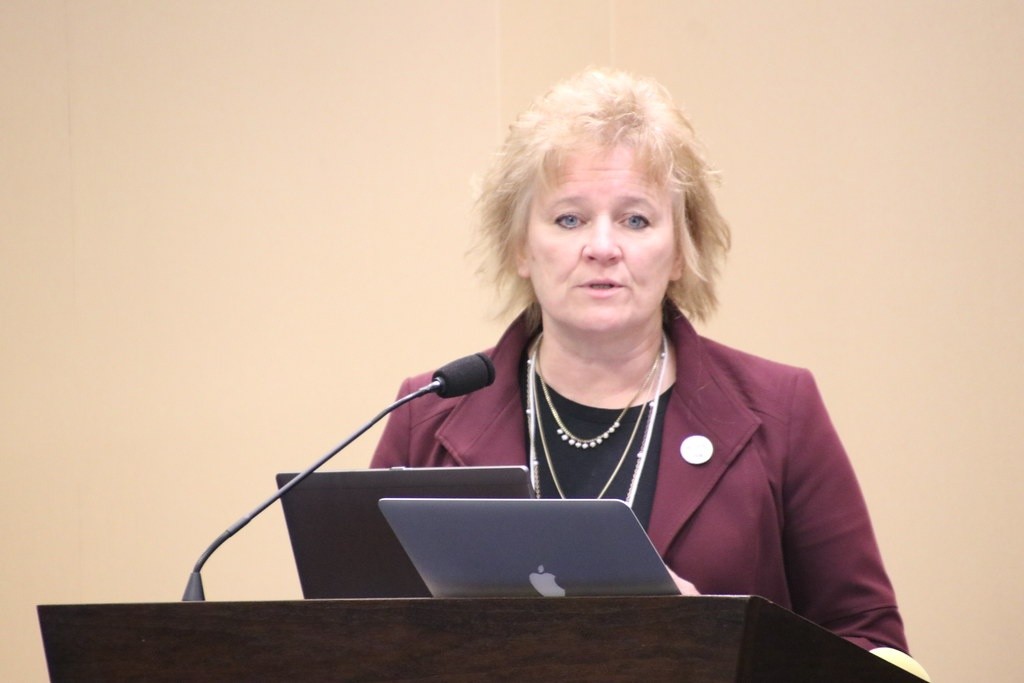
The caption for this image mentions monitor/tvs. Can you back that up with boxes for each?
[275,465,537,599]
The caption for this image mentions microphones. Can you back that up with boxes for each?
[181,352,498,604]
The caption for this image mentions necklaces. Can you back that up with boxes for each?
[525,329,669,506]
[537,346,661,449]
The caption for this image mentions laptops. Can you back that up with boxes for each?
[377,497,684,599]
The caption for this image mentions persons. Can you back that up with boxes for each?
[369,70,930,683]
